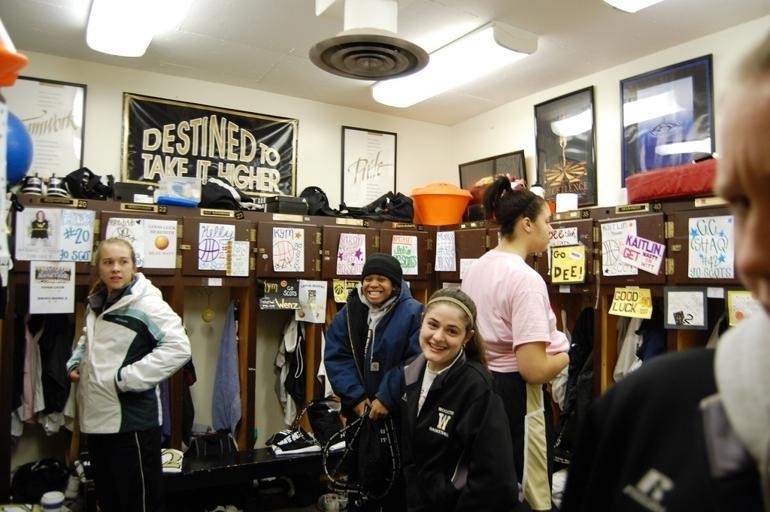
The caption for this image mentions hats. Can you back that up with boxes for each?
[362,253,403,288]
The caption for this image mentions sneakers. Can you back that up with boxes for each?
[16,176,42,197]
[48,178,72,193]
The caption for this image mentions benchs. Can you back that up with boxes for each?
[169,435,348,512]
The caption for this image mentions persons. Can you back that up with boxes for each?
[25,210,52,249]
[561,34,770,511]
[65,238,192,512]
[324,254,427,512]
[460,176,569,512]
[404,287,518,512]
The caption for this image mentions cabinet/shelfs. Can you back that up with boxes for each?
[431,195,764,441]
[3,194,431,512]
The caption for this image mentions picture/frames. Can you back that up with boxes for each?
[619,53,716,189]
[458,149,527,189]
[340,125,397,210]
[121,91,300,199]
[0,75,87,178]
[533,85,598,209]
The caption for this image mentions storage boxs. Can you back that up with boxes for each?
[113,182,154,204]
[265,196,307,215]
[157,174,202,208]
